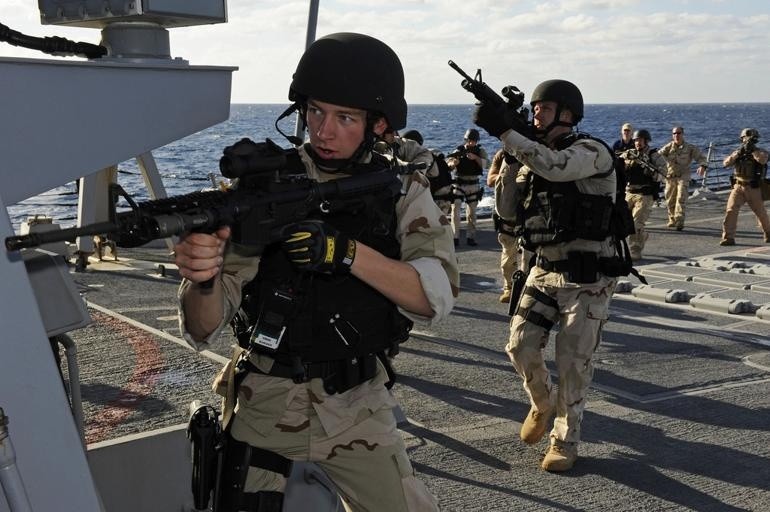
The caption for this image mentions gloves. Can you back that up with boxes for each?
[472,104,511,140]
[279,219,356,278]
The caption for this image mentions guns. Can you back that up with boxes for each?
[443,145,481,164]
[449,60,547,164]
[5,140,403,301]
[740,134,759,161]
[624,147,669,179]
[188,399,218,511]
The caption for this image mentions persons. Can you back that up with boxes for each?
[473,77,622,472]
[612,122,635,154]
[173,33,459,511]
[400,130,452,223]
[487,147,532,301]
[620,129,667,263]
[658,126,709,232]
[447,129,489,247]
[720,127,770,245]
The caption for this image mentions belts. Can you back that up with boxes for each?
[246,360,342,383]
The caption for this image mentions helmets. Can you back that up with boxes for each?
[631,130,652,142]
[740,127,762,140]
[403,129,424,145]
[289,32,407,132]
[530,80,584,119]
[463,128,481,142]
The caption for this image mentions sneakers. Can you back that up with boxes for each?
[541,436,577,471]
[763,231,770,245]
[521,406,555,443]
[667,219,685,231]
[500,287,511,304]
[630,250,641,261]
[719,239,736,247]
